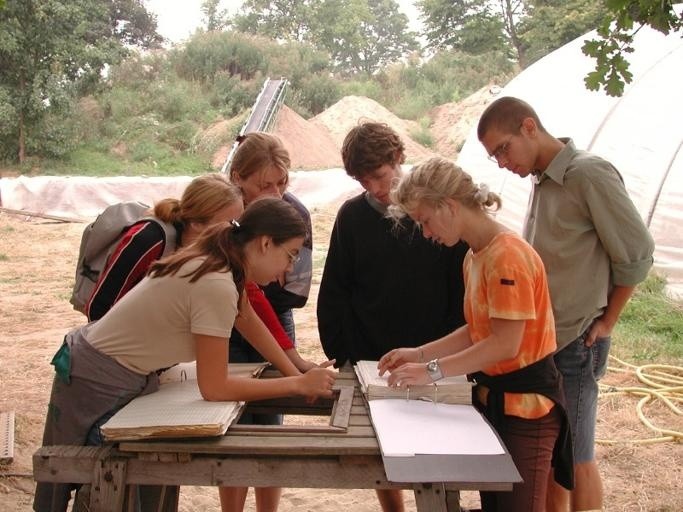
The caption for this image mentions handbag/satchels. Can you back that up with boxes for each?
[68,199,178,316]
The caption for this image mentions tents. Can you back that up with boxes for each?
[456,4,682,308]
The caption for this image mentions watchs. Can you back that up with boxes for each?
[426,358,443,382]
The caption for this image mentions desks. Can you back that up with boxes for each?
[32,367,514,512]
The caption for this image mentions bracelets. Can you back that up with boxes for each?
[416,345,425,361]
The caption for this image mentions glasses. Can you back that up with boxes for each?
[485,121,526,164]
[274,240,302,267]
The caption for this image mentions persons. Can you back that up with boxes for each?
[218,134,313,512]
[376,157,575,512]
[317,117,468,511]
[85,174,337,373]
[32,197,336,512]
[477,97,656,512]
